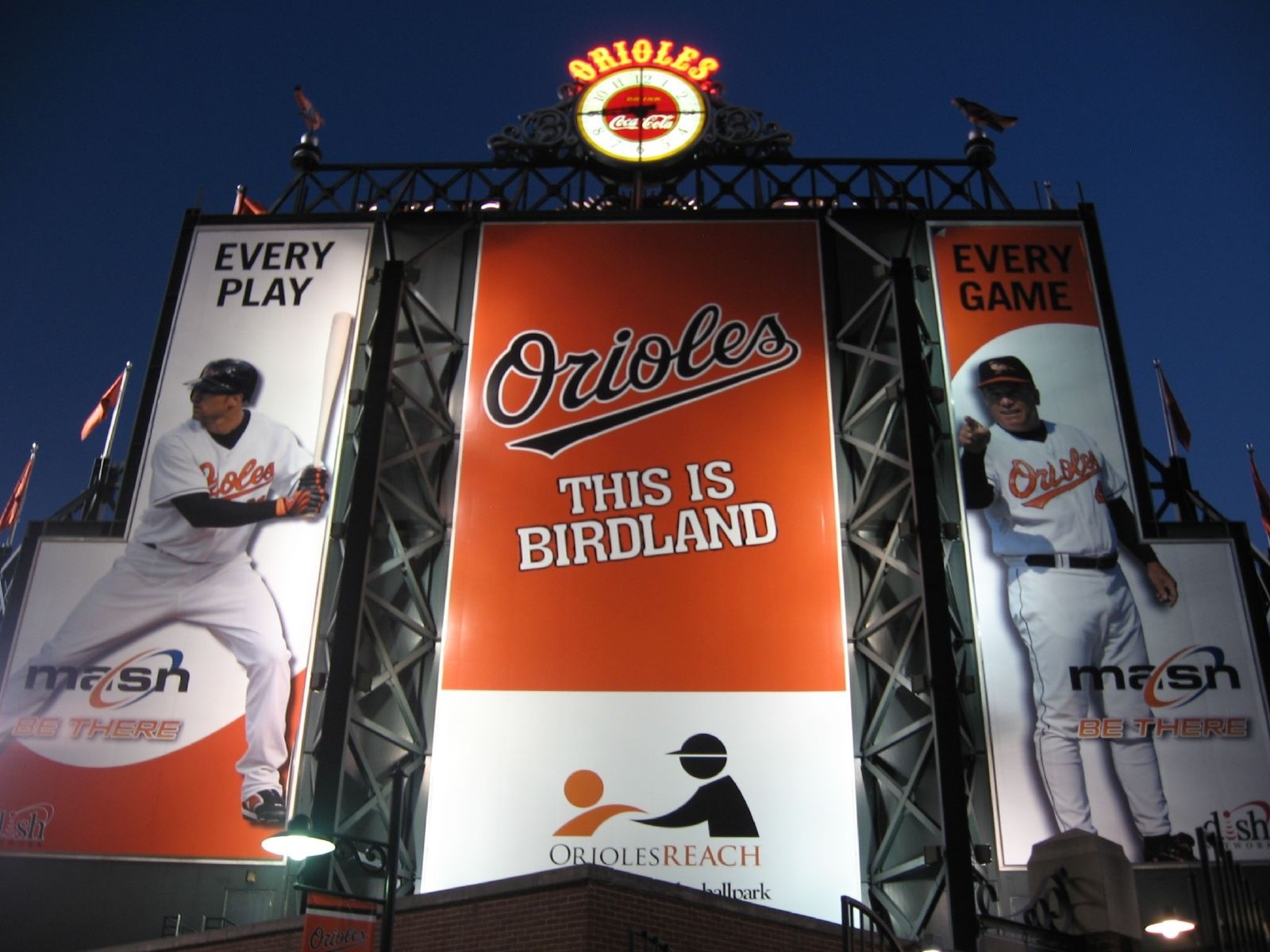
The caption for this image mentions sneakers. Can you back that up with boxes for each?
[240,788,286,824]
[1142,833,1199,865]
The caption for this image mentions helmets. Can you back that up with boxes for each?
[182,358,258,404]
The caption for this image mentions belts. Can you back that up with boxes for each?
[1024,550,1118,570]
[145,542,158,549]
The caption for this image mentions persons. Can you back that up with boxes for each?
[0,359,327,824]
[955,355,1198,864]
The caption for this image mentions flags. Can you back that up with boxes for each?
[1248,457,1270,540]
[0,460,33,531]
[81,373,123,440]
[1160,373,1192,452]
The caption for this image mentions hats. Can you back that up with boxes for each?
[976,355,1033,390]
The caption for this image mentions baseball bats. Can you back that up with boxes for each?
[304,314,354,519]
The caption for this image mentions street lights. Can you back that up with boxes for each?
[260,766,407,952]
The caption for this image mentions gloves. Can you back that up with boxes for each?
[277,467,330,520]
